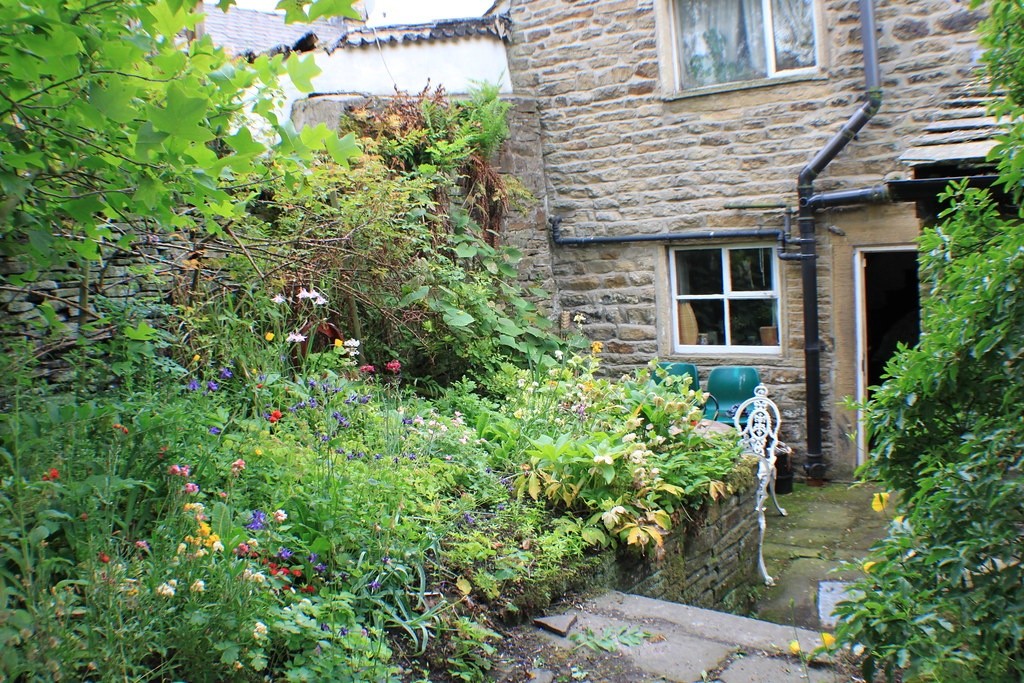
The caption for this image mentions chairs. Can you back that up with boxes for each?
[650,363,760,426]
[732,382,788,587]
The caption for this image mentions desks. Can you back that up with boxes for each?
[694,418,735,438]
